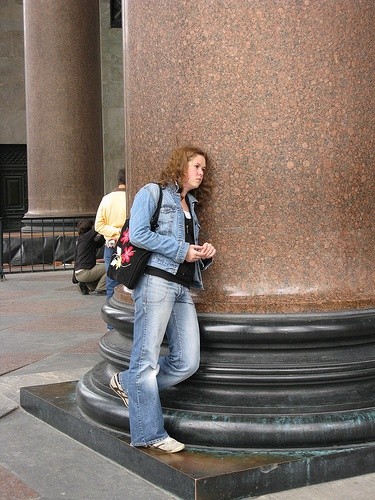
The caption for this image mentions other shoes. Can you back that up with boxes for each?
[97,290,107,296]
[79,283,89,296]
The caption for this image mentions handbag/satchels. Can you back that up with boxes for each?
[106,181,163,290]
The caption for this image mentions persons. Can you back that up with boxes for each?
[94,167,126,333]
[75,218,108,296]
[109,147,217,453]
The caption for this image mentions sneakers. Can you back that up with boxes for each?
[148,435,186,454]
[109,372,132,409]
